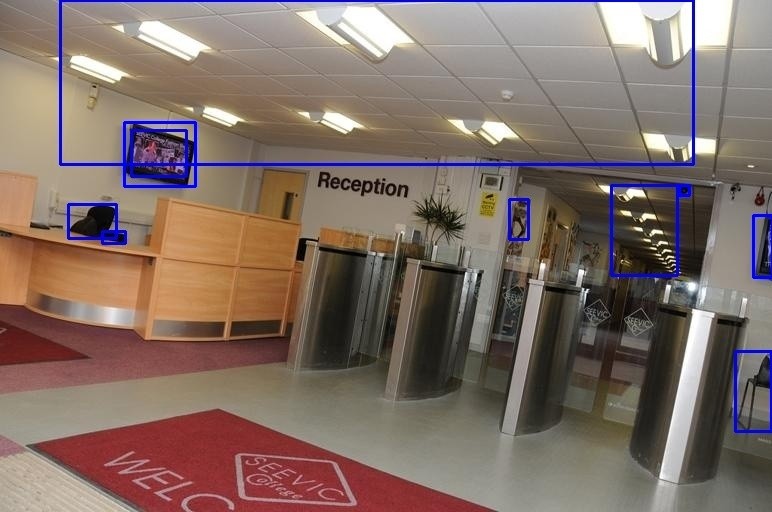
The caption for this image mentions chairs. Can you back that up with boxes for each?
[736,351,772,432]
[68,205,116,239]
[102,232,125,243]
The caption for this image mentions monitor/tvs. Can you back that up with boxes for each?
[754,215,772,278]
[125,123,196,187]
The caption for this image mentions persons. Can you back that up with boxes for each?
[509,199,528,239]
[131,131,187,176]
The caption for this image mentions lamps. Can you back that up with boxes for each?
[187,104,247,129]
[443,118,519,146]
[596,2,692,69]
[299,110,363,134]
[612,185,678,275]
[642,131,693,163]
[60,54,134,83]
[295,5,412,64]
[112,21,212,65]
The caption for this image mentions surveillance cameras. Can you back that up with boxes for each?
[679,185,689,195]
